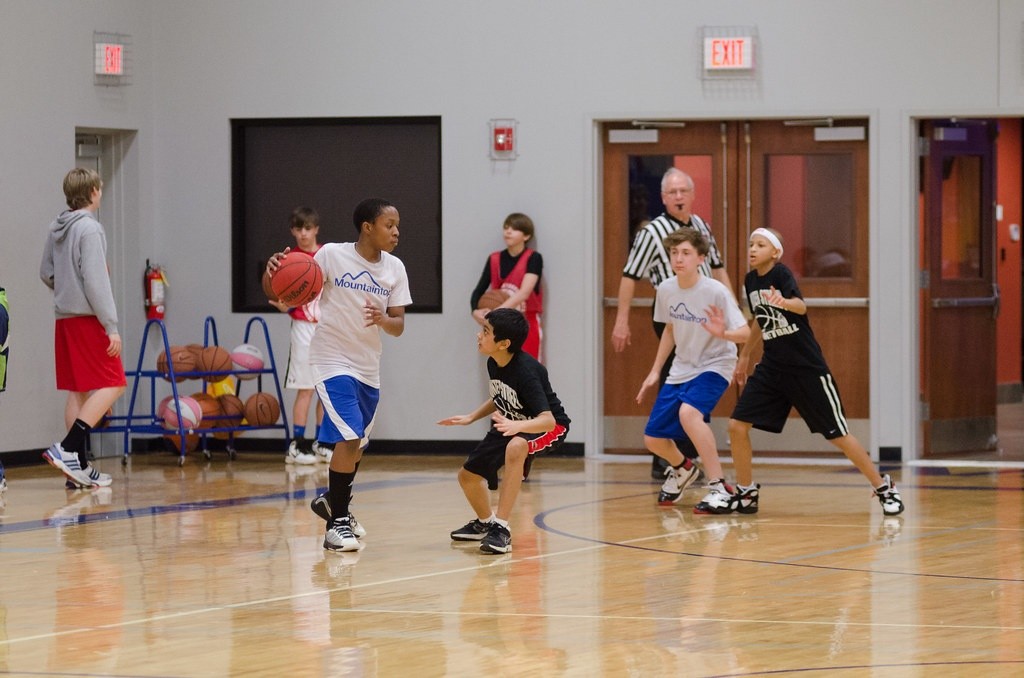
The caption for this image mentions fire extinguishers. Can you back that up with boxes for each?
[146,264,166,320]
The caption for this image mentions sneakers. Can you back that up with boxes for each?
[734,483,760,514]
[44,444,112,490]
[692,479,739,515]
[658,506,700,546]
[481,553,513,586]
[285,463,330,486]
[284,439,333,465]
[479,522,513,554]
[731,512,759,543]
[692,513,732,543]
[450,519,492,541]
[657,459,700,506]
[877,516,904,548]
[49,487,113,525]
[310,494,366,554]
[324,541,366,580]
[872,474,905,516]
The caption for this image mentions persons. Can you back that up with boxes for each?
[0,287,10,496]
[263,198,413,552]
[470,213,545,363]
[268,205,335,465]
[437,308,571,552]
[39,167,129,491]
[799,247,851,276]
[611,169,740,483]
[636,226,751,514]
[709,228,904,517]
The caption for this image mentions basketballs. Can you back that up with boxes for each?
[216,395,245,428]
[245,393,280,426]
[184,344,203,380]
[189,393,219,428]
[262,269,280,302]
[158,395,203,430]
[92,406,113,428]
[157,346,195,383]
[167,432,199,454]
[230,344,264,380]
[478,290,510,311]
[197,346,233,383]
[270,252,324,307]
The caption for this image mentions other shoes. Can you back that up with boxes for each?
[651,455,705,481]
